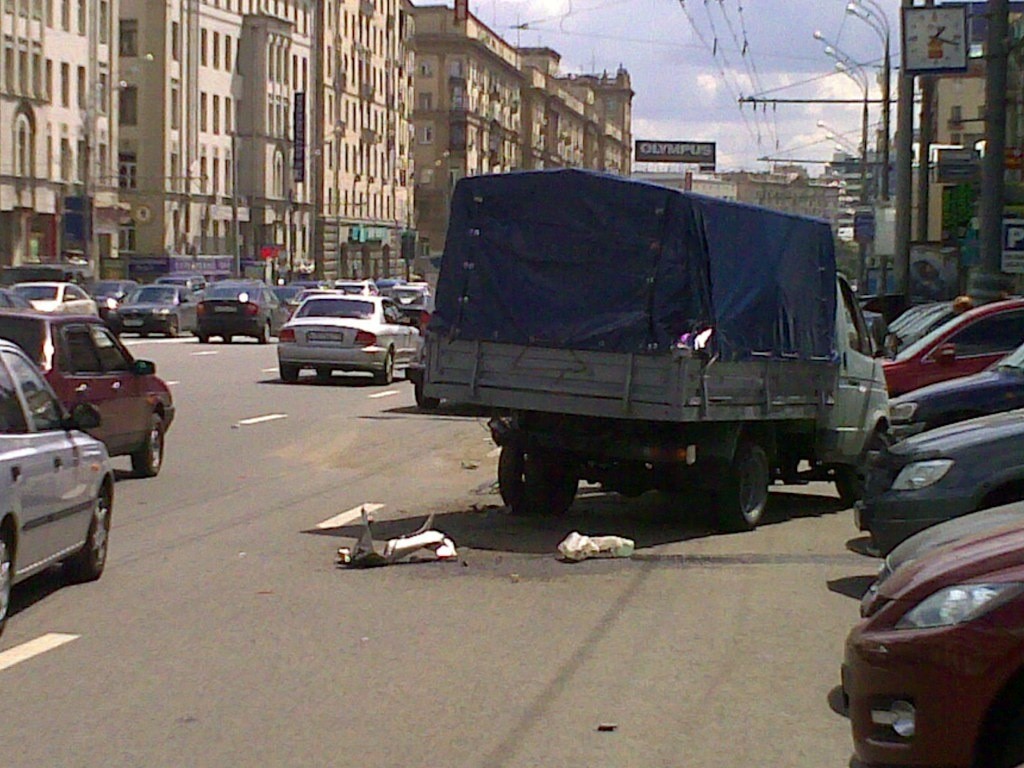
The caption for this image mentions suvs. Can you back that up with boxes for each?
[155,273,208,302]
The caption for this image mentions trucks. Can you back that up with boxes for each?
[422,169,893,531]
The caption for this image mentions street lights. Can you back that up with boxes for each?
[845,1,890,299]
[812,31,869,295]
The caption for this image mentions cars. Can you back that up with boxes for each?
[85,280,139,324]
[195,280,293,344]
[877,298,1024,398]
[270,277,430,331]
[0,310,176,478]
[0,288,35,312]
[8,279,100,315]
[0,340,116,640]
[882,344,1024,445]
[845,289,1000,360]
[855,409,1024,560]
[108,285,200,337]
[278,294,425,387]
[828,501,1023,768]
